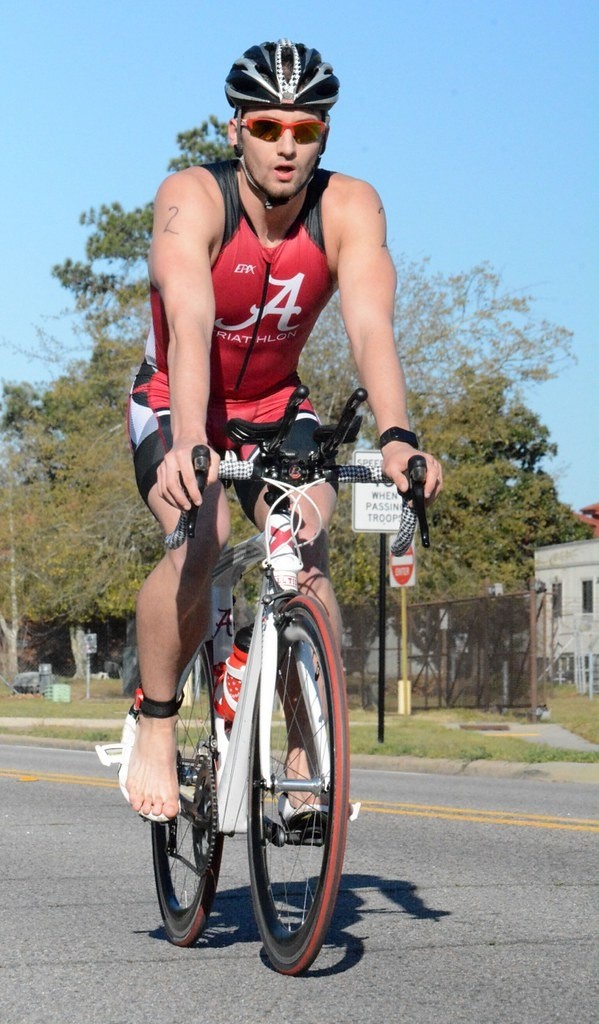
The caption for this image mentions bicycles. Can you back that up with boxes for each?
[94,386,432,976]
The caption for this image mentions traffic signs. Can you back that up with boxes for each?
[388,534,416,588]
[84,633,97,654]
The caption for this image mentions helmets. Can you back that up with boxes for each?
[224,38,341,111]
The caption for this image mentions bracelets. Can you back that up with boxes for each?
[137,687,185,718]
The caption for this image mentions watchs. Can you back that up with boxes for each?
[375,426,421,453]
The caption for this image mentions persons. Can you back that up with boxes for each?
[117,35,443,822]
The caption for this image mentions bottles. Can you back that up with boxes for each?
[214,624,254,720]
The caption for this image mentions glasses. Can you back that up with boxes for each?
[235,115,326,145]
